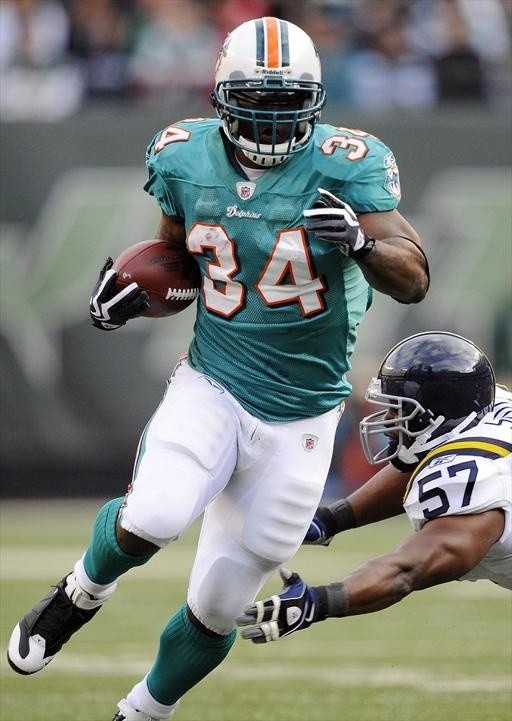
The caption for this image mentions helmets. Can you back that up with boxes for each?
[375,330,496,472]
[206,14,327,171]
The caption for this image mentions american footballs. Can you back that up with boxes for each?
[111,239,199,320]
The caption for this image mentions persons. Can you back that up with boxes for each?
[7,14,433,720]
[232,327,512,647]
[0,1,511,123]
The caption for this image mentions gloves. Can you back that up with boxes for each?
[299,187,375,264]
[87,253,154,335]
[234,566,329,647]
[299,506,339,547]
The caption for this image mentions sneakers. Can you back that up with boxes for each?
[6,567,108,677]
